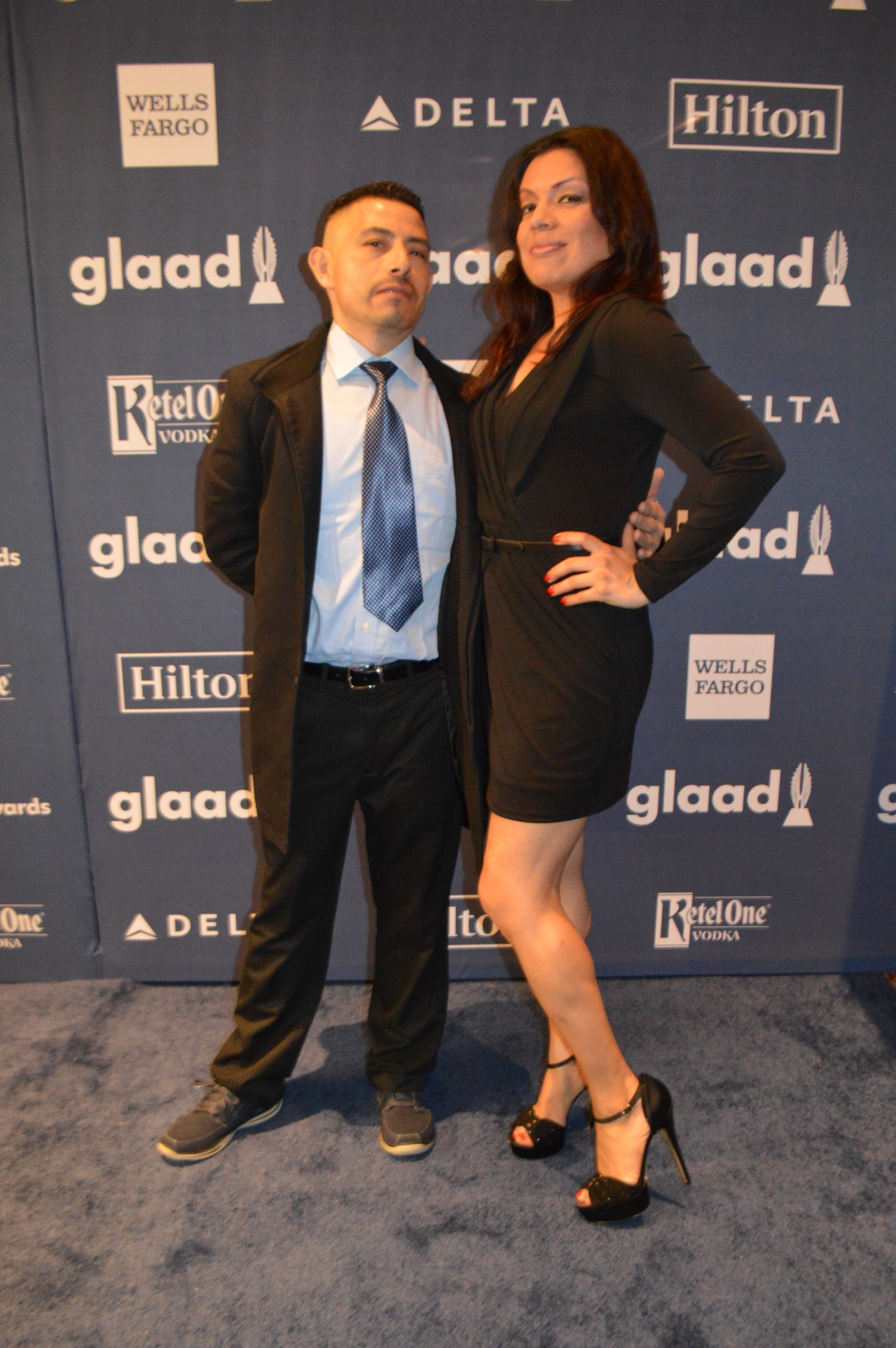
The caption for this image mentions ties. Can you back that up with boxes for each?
[362,358,428,629]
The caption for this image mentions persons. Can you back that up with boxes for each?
[154,179,666,1165]
[457,123,790,1221]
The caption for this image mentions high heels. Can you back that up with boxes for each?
[508,1048,589,1160]
[574,1072,693,1222]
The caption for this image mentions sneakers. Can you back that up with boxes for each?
[154,1073,290,1164]
[376,1081,435,1162]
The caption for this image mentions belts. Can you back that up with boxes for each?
[297,659,448,691]
[477,533,633,547]
